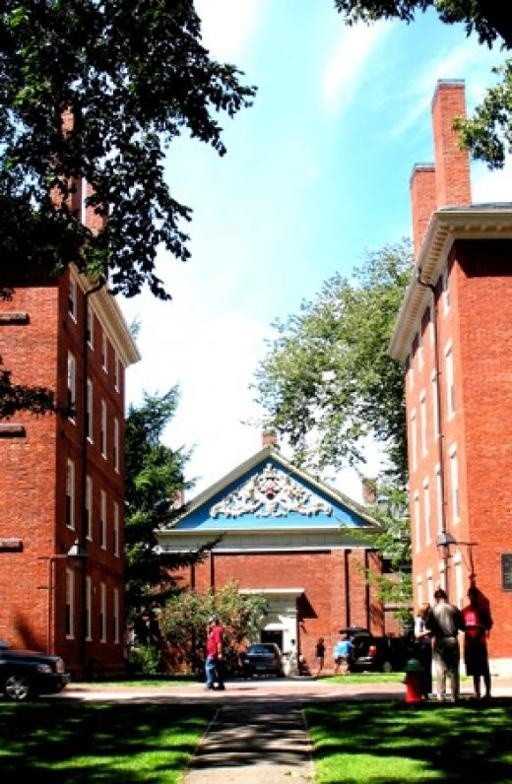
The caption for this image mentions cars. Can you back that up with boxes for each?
[243,643,288,678]
[339,627,420,673]
[0,641,71,702]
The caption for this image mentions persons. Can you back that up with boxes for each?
[333,633,356,673]
[313,637,324,679]
[414,586,493,701]
[204,615,225,689]
